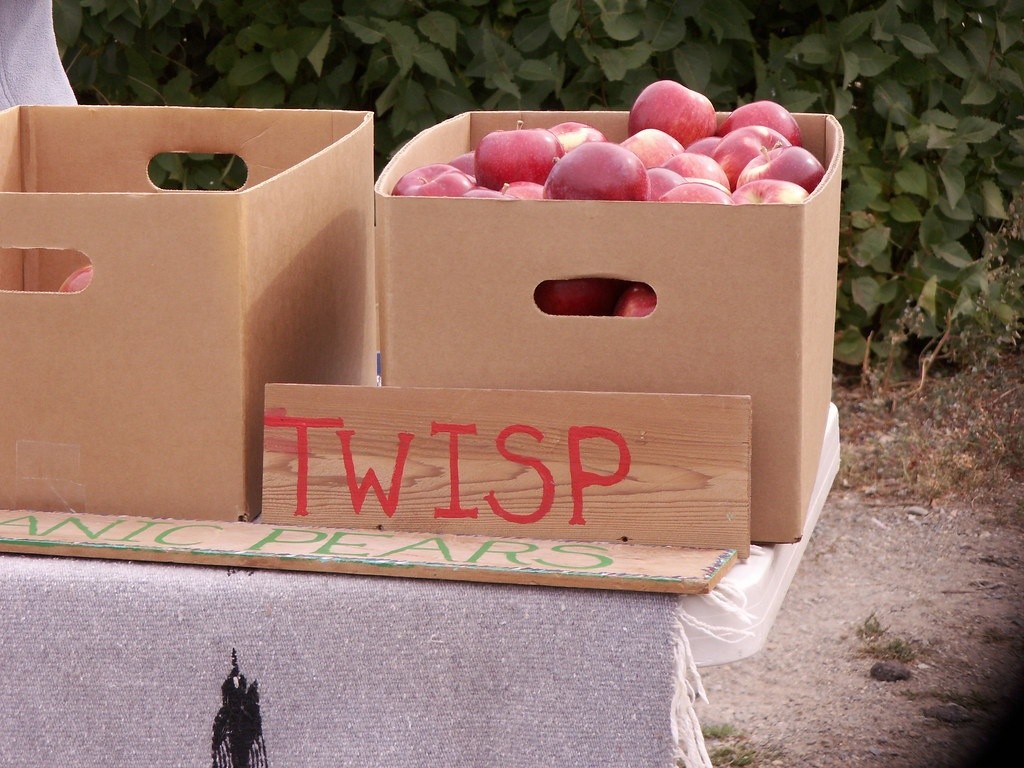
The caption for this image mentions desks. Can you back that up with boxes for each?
[1,403,845,768]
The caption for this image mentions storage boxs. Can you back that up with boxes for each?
[374,111,845,543]
[0,103,374,524]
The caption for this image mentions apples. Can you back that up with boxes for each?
[388,80,824,317]
[57,265,93,294]
